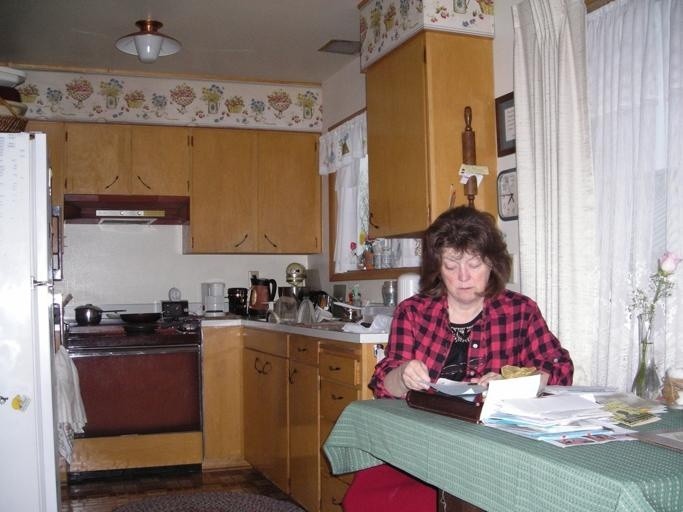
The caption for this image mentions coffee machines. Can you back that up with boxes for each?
[199,281,225,319]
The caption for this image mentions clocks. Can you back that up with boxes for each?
[496,168,520,221]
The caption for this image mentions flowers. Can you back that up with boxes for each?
[626,250,682,321]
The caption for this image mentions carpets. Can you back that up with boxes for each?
[110,490,308,512]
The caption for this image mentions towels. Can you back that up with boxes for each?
[54,346,87,467]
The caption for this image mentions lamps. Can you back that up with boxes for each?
[113,17,181,64]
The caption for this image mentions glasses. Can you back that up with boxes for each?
[440,357,485,377]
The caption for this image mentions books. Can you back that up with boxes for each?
[406,372,683,452]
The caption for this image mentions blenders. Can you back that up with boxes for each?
[278,262,311,308]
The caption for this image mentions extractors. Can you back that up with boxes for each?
[63,199,187,227]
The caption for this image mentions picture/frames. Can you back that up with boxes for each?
[494,91,515,158]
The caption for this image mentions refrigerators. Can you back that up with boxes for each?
[0,133,62,512]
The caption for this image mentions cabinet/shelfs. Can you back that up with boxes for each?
[242,327,288,495]
[182,128,321,255]
[25,121,64,254]
[67,124,189,196]
[319,339,362,512]
[288,335,321,512]
[365,31,498,242]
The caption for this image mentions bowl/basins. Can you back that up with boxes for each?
[331,300,365,322]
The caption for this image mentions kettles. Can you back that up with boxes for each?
[248,274,277,315]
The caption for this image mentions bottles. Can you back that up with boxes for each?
[365,249,374,270]
[227,286,248,315]
[373,248,383,269]
[381,247,392,268]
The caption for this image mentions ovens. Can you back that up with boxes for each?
[65,340,202,439]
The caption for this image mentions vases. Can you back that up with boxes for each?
[630,314,661,400]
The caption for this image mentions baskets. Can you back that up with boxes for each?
[0,98,28,132]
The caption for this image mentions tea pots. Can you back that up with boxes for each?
[174,311,201,333]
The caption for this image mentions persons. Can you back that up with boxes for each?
[340,204,575,511]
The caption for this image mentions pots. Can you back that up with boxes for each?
[74,303,127,325]
[119,311,164,322]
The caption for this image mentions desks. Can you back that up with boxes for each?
[321,385,682,512]
